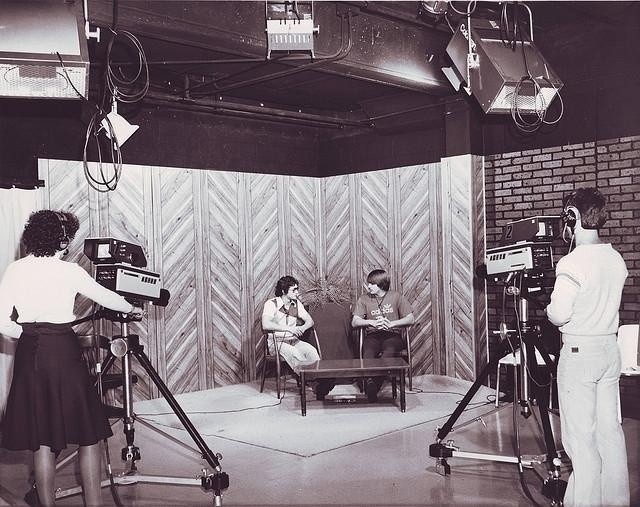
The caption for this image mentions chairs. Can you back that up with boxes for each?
[616,322,640,426]
[493,319,564,412]
[359,325,412,389]
[260,319,323,399]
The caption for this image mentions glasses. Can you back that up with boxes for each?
[288,286,299,293]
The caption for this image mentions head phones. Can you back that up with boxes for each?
[560,189,582,232]
[53,209,70,251]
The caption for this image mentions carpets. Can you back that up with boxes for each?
[117,370,506,461]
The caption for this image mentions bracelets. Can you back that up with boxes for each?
[131,305,135,312]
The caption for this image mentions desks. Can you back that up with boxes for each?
[300,356,410,416]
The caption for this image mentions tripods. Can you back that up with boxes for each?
[24,325,230,505]
[428,316,569,506]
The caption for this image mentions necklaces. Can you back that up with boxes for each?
[373,292,386,318]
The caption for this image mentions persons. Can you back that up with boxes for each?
[351,269,416,402]
[544,186,633,507]
[0,208,146,506]
[261,275,337,401]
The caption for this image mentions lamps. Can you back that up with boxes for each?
[264,1,320,61]
[417,1,449,21]
[95,86,142,152]
